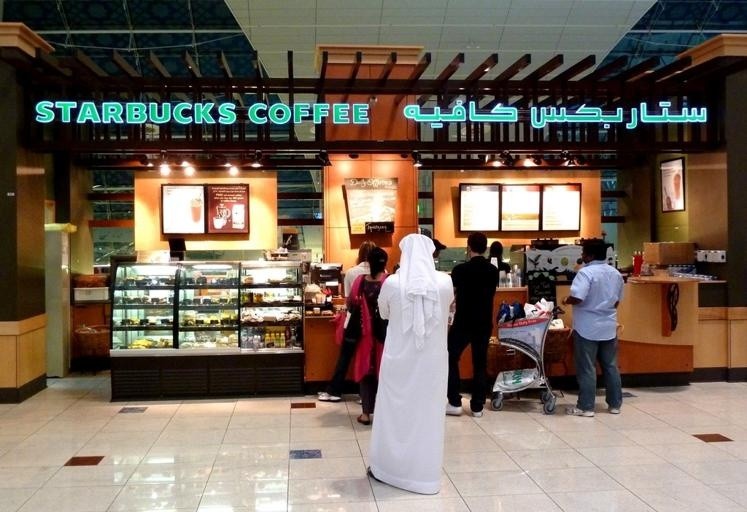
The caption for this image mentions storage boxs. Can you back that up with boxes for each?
[643,241,697,266]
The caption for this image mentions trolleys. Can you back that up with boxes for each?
[491,307,566,414]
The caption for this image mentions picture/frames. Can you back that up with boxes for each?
[457,181,500,233]
[539,184,583,231]
[501,184,542,230]
[160,182,205,234]
[205,183,250,233]
[660,156,683,212]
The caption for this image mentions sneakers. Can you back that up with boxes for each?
[353,393,361,403]
[472,407,483,416]
[565,406,594,417]
[609,406,620,414]
[446,403,462,414]
[319,391,341,402]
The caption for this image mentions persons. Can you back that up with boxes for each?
[367,234,456,494]
[488,241,511,273]
[346,248,391,424]
[318,241,387,404]
[431,239,446,258]
[562,239,622,415]
[448,233,499,417]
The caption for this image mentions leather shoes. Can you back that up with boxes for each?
[357,413,370,425]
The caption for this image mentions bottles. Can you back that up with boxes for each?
[241,327,262,350]
[667,263,697,276]
[274,333,280,349]
[264,334,270,348]
[631,250,645,276]
[280,333,285,349]
[290,329,296,347]
[270,333,275,348]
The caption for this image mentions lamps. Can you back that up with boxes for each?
[566,156,576,168]
[500,155,514,166]
[533,158,544,166]
[251,152,263,167]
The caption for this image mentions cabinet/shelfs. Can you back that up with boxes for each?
[108,260,305,401]
[307,267,722,396]
